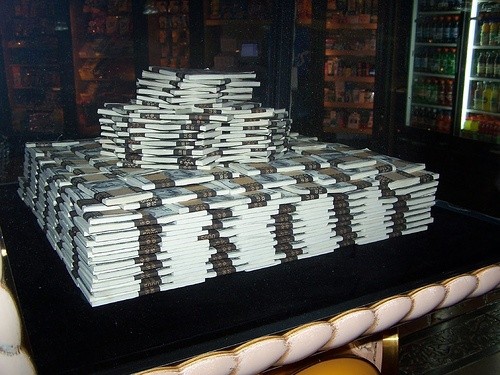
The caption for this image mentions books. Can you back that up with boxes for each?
[16,64,440,309]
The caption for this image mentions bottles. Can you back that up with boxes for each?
[413,11,499,139]
